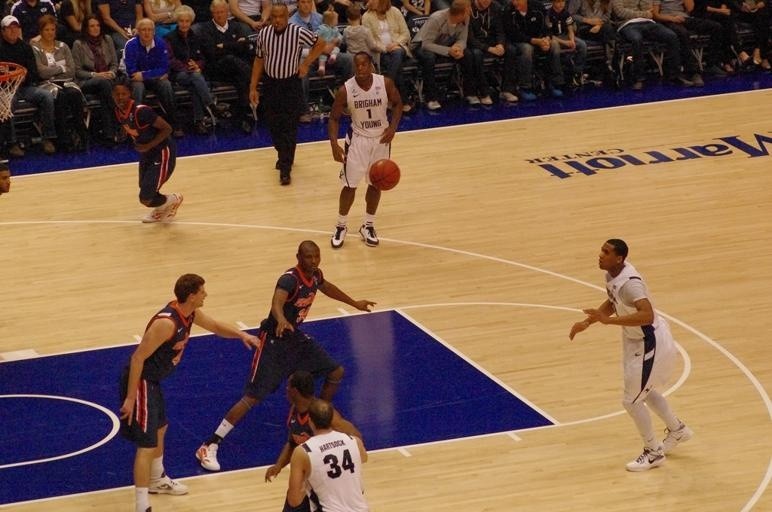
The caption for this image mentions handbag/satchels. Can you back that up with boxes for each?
[85,107,107,146]
[63,116,104,152]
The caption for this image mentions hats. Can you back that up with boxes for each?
[1,14,19,28]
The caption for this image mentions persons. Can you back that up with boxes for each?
[196,240,380,472]
[117,273,261,512]
[0,1,272,156]
[566,239,693,472]
[286,401,373,511]
[264,372,365,512]
[328,51,403,248]
[249,3,327,185]
[111,83,183,224]
[1,164,10,193]
[285,2,771,124]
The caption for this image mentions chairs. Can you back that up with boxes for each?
[0,13,772,150]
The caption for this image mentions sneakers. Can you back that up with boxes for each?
[674,73,705,87]
[428,86,564,110]
[359,224,379,247]
[276,159,290,185]
[626,447,665,471]
[142,191,183,224]
[632,81,642,90]
[10,146,25,157]
[331,224,347,248]
[663,424,692,453]
[195,440,220,472]
[43,141,56,153]
[148,472,189,495]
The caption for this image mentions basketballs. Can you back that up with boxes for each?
[369,161,400,191]
